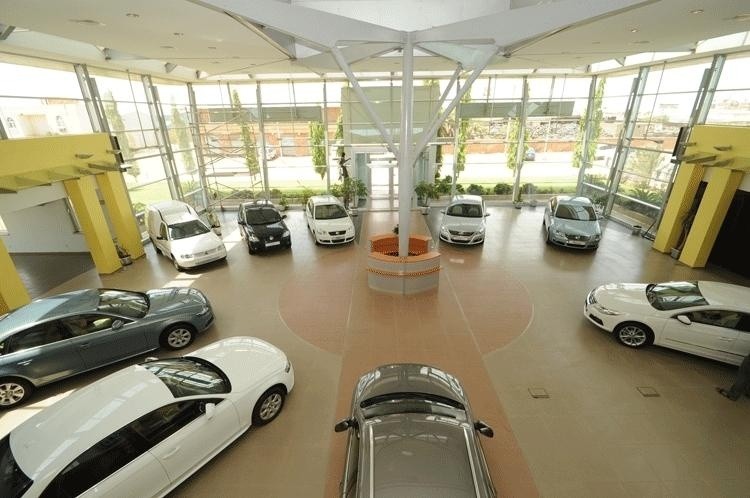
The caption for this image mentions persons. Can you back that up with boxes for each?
[716,354,750,401]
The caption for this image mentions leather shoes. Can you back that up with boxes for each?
[722,391,731,398]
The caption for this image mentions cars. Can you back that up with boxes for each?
[0,332,297,498]
[542,193,605,253]
[576,272,750,367]
[437,192,490,247]
[1,281,218,409]
[324,359,504,497]
[524,145,536,161]
[304,192,357,250]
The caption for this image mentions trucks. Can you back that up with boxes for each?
[142,198,228,270]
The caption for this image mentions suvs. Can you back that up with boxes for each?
[235,199,292,256]
